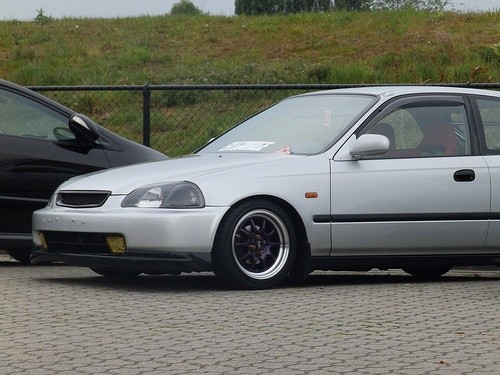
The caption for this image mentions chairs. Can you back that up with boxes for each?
[364,122,396,160]
[412,121,457,156]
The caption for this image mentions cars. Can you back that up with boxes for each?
[0,77,170,264]
[31,86,499,291]
